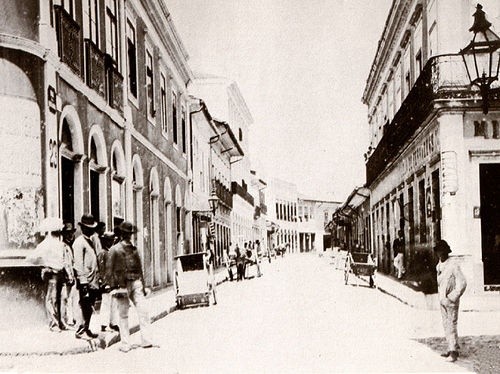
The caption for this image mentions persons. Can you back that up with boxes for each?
[105,220,161,352]
[71,215,104,341]
[204,228,406,304]
[433,240,467,362]
[34,216,76,334]
[61,223,81,328]
[79,216,132,328]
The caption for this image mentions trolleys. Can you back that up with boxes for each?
[344,251,378,289]
[172,252,217,309]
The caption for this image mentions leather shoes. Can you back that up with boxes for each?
[48,319,152,353]
[441,351,459,362]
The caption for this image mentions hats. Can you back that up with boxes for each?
[433,239,452,253]
[45,216,138,235]
